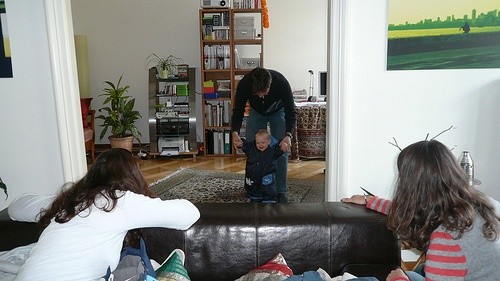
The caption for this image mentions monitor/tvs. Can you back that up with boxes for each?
[320,72,327,101]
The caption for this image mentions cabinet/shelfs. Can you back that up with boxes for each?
[149,66,198,162]
[199,9,263,157]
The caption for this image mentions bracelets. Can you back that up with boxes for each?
[284,134,291,138]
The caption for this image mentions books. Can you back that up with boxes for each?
[203,79,231,99]
[231,0,261,9]
[202,12,230,41]
[205,129,232,155]
[205,100,231,127]
[204,45,230,70]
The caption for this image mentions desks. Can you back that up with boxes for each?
[292,105,326,160]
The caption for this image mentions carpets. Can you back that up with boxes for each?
[148,166,325,204]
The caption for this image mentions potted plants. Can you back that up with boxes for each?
[144,51,184,79]
[97,74,143,153]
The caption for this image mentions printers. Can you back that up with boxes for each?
[158,136,185,156]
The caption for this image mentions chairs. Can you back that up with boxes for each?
[81,97,95,164]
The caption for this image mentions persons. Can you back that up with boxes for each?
[232,67,296,203]
[14,148,201,281]
[234,129,290,203]
[341,140,500,281]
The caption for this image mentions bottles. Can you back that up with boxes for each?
[457,151,474,187]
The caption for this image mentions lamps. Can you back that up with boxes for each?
[308,70,315,102]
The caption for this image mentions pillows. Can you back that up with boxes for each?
[152,252,190,281]
[234,252,294,281]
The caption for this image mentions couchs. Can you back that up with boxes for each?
[0,203,401,281]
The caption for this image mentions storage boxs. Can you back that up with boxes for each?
[217,80,230,90]
[177,85,188,96]
[234,29,256,39]
[204,82,214,88]
[204,93,218,99]
[204,87,216,93]
[240,58,260,68]
[217,89,230,98]
[234,17,254,29]
